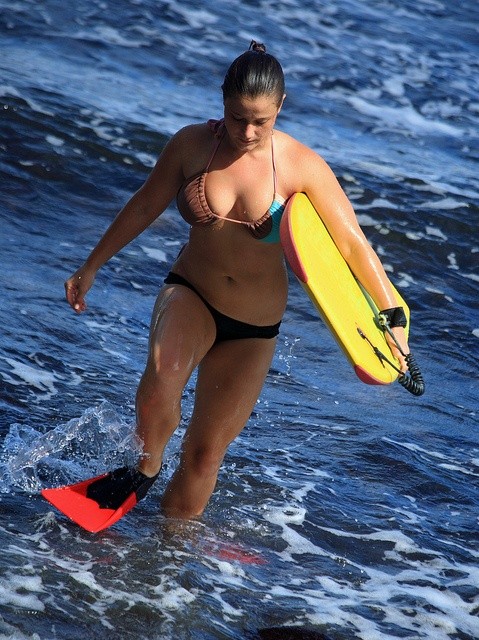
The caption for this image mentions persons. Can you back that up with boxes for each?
[61,37,413,518]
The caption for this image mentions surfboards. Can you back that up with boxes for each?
[279,193,410,385]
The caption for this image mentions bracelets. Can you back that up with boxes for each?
[377,307,407,329]
